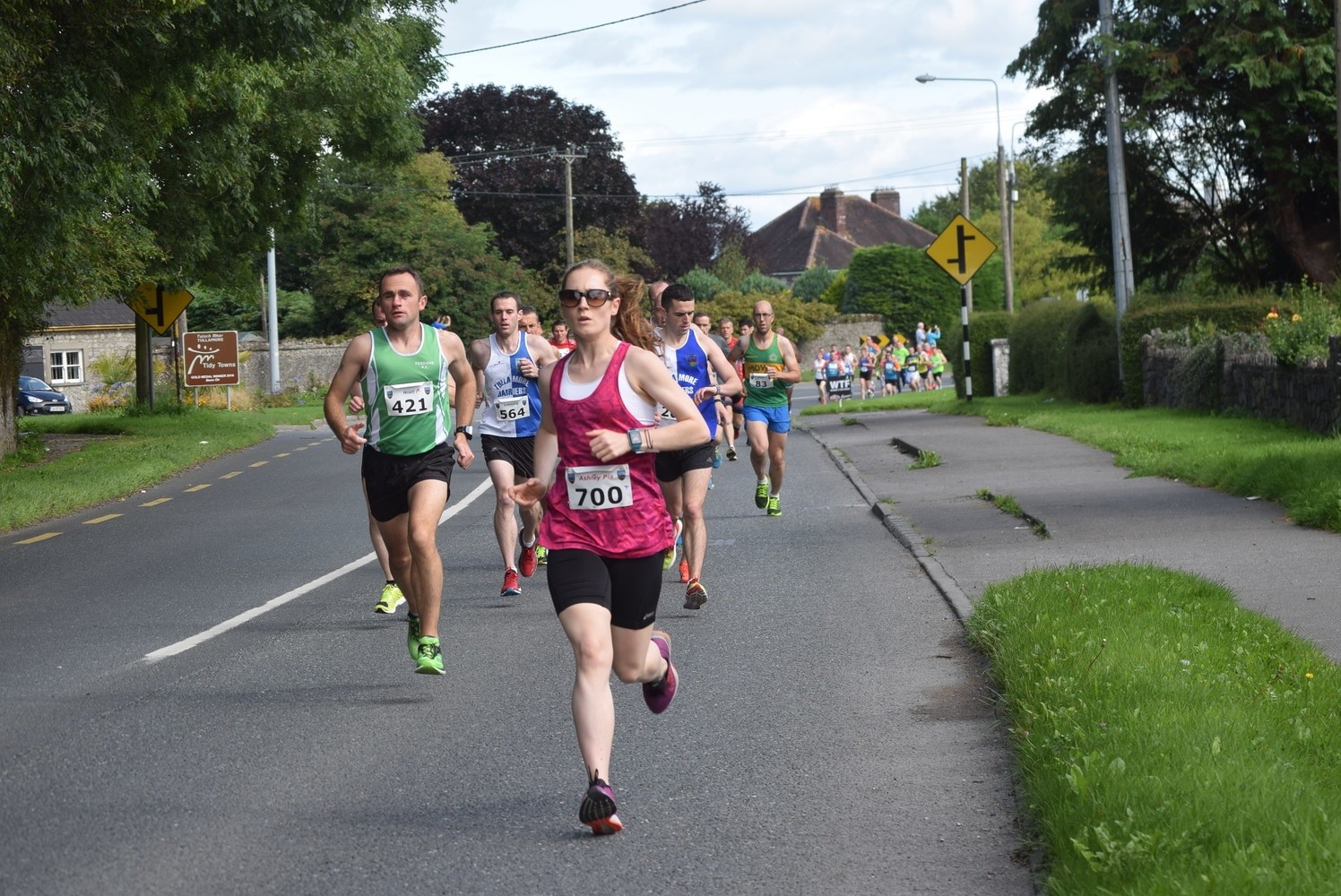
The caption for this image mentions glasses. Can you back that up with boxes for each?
[560,289,616,308]
[753,314,773,320]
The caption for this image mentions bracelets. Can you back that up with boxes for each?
[644,429,653,449]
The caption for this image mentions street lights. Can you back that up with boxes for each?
[916,74,1016,310]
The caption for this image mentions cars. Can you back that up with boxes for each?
[15,376,73,415]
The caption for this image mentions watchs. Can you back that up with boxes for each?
[453,425,473,441]
[627,428,642,452]
[708,382,720,395]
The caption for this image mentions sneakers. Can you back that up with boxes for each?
[579,766,623,834]
[767,496,782,516]
[733,425,739,439]
[662,519,683,571]
[642,631,678,714]
[684,578,707,610]
[679,562,690,583]
[534,544,549,565]
[713,448,722,468]
[754,474,770,509]
[374,583,406,614]
[727,447,737,461]
[415,635,446,675]
[500,566,522,597]
[675,535,683,546]
[407,611,421,661]
[708,475,715,490]
[517,526,538,578]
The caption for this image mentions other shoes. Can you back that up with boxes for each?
[747,438,751,446]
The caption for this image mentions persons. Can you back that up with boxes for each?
[349,280,802,615]
[324,267,475,676]
[506,259,712,834]
[810,322,946,402]
